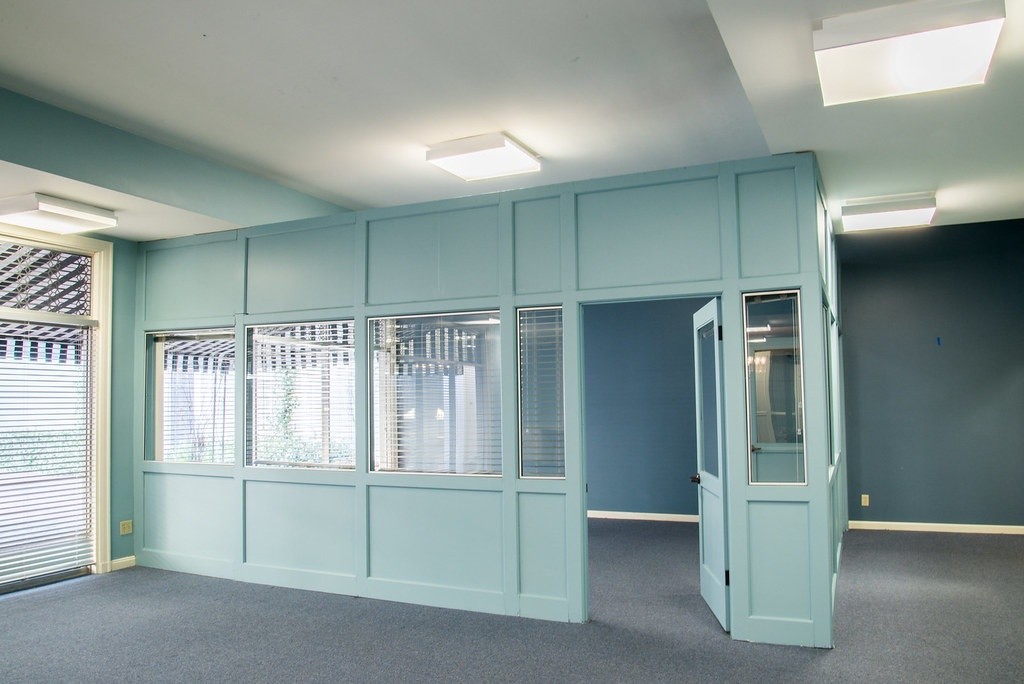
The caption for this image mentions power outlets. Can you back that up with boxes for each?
[861,494,869,506]
[119,520,133,535]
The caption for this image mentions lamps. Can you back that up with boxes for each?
[841,196,936,232]
[426,128,541,183]
[0,192,118,236]
[813,0,1007,106]
